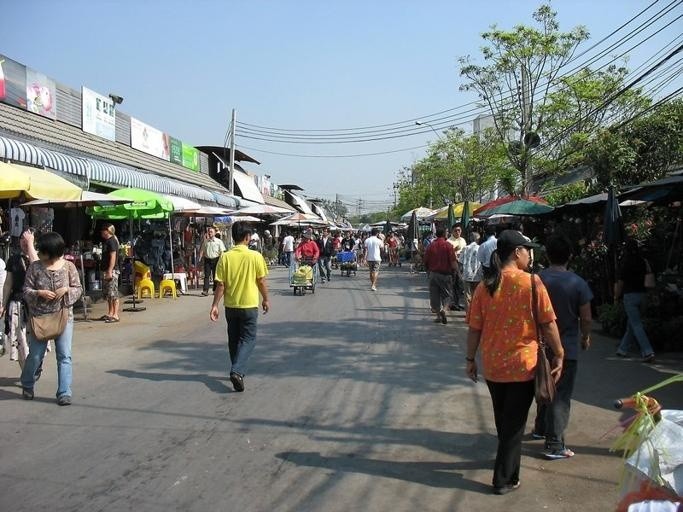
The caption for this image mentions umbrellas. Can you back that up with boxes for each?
[174,206,233,231]
[0,161,83,209]
[19,189,134,207]
[602,185,627,252]
[556,190,638,211]
[479,199,555,228]
[472,194,550,215]
[85,188,174,240]
[163,195,201,281]
[402,206,433,218]
[616,175,683,201]
[269,212,328,229]
[238,204,295,230]
[461,199,469,237]
[435,199,482,219]
[448,204,456,228]
[406,211,419,258]
[428,205,448,217]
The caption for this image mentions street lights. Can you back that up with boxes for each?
[413,121,450,151]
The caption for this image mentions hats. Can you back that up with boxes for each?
[485,225,496,234]
[303,232,311,239]
[496,229,538,262]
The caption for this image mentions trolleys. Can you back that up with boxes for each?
[386,243,402,269]
[289,260,318,296]
[337,252,358,276]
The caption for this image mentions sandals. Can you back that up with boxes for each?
[99,315,110,320]
[105,316,119,323]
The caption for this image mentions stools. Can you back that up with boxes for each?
[163,272,189,295]
[137,279,155,300]
[158,280,176,299]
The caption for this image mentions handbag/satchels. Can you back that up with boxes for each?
[531,273,557,404]
[28,257,69,340]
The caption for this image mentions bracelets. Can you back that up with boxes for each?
[466,356,475,361]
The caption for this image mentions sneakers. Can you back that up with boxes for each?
[202,290,208,295]
[641,354,654,362]
[434,318,441,323]
[58,395,72,405]
[492,481,520,495]
[440,310,448,324]
[616,351,628,357]
[322,279,325,283]
[372,286,376,290]
[540,445,574,458]
[328,276,331,281]
[23,388,34,400]
[230,371,245,392]
[533,429,546,438]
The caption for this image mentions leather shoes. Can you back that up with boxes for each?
[450,304,462,310]
[458,304,464,309]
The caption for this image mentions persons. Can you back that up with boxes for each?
[465,229,564,498]
[249,228,260,250]
[614,239,656,363]
[316,228,334,283]
[532,232,592,461]
[379,231,434,269]
[281,231,294,268]
[445,224,466,312]
[21,233,83,406]
[263,229,276,250]
[0,228,51,380]
[210,221,271,392]
[199,227,226,297]
[329,227,371,267]
[458,232,481,323]
[423,227,462,325]
[363,228,384,291]
[478,228,498,278]
[98,223,120,324]
[295,234,320,290]
